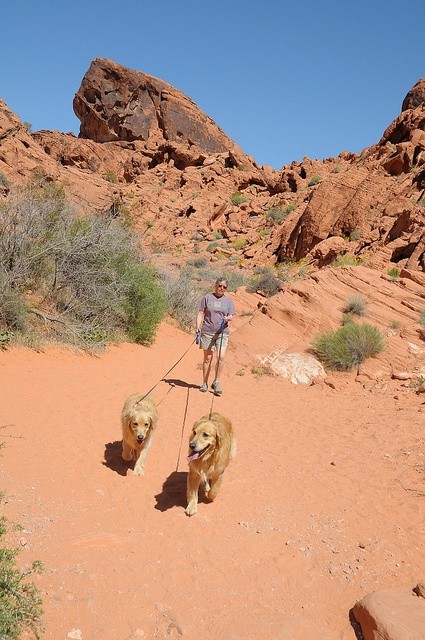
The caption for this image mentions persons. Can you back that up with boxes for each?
[195,277,235,395]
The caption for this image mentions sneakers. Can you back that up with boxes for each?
[211,380,222,395]
[199,384,208,392]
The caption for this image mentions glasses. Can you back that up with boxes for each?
[218,284,227,289]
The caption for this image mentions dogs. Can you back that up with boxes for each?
[184,412,235,517]
[121,393,159,477]
